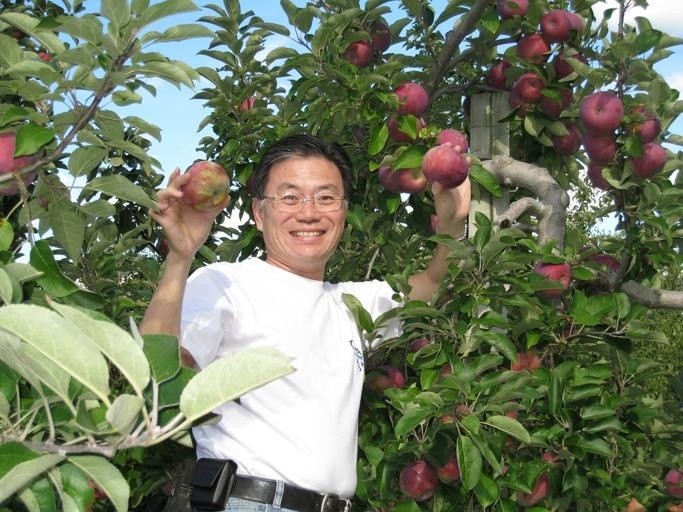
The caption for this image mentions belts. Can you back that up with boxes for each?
[229,473,353,512]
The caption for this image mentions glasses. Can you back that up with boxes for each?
[257,190,348,214]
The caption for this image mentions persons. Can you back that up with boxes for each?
[134,132,473,512]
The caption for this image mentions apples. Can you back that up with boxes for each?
[240,0,682,512]
[158,237,169,261]
[0,132,37,196]
[85,480,107,501]
[182,160,230,212]
[37,49,50,62]
[9,29,24,37]
